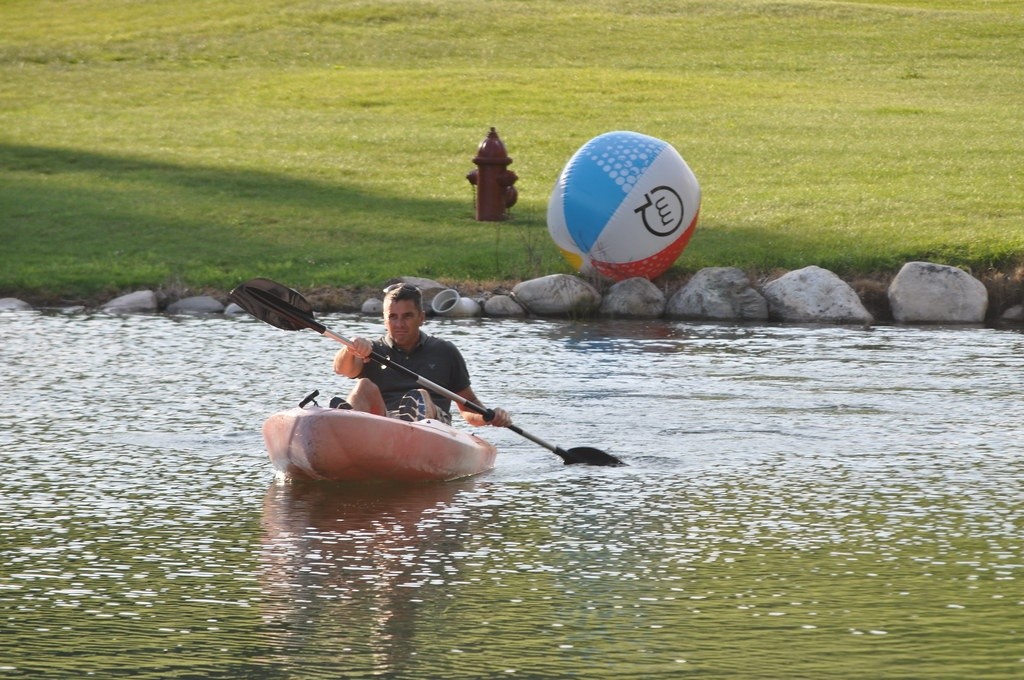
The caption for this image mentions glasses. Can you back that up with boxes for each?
[383,283,423,311]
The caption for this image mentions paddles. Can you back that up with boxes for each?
[225,276,632,469]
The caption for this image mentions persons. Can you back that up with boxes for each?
[329,282,512,428]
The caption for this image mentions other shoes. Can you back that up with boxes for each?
[399,389,425,422]
[330,396,354,410]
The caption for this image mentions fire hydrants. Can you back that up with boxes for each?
[466,127,518,221]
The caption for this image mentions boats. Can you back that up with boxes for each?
[262,390,497,479]
